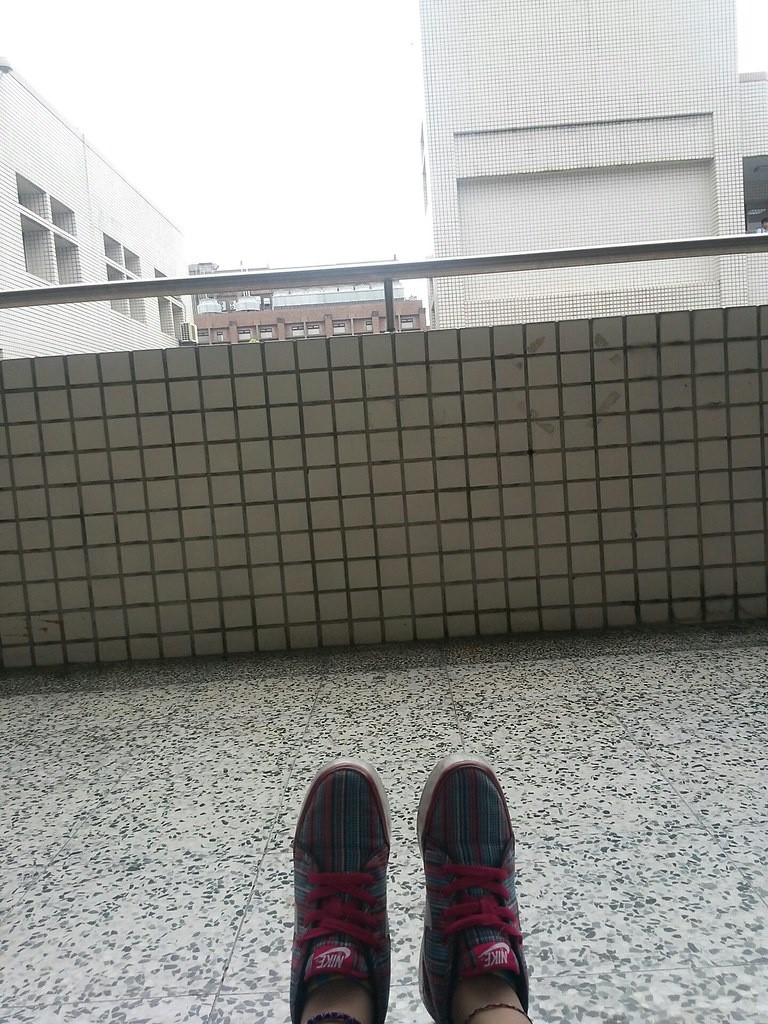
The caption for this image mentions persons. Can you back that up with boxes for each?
[756,218,768,234]
[287,756,534,1024]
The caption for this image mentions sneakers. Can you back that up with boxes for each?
[416,753,529,1024]
[290,757,392,1024]
